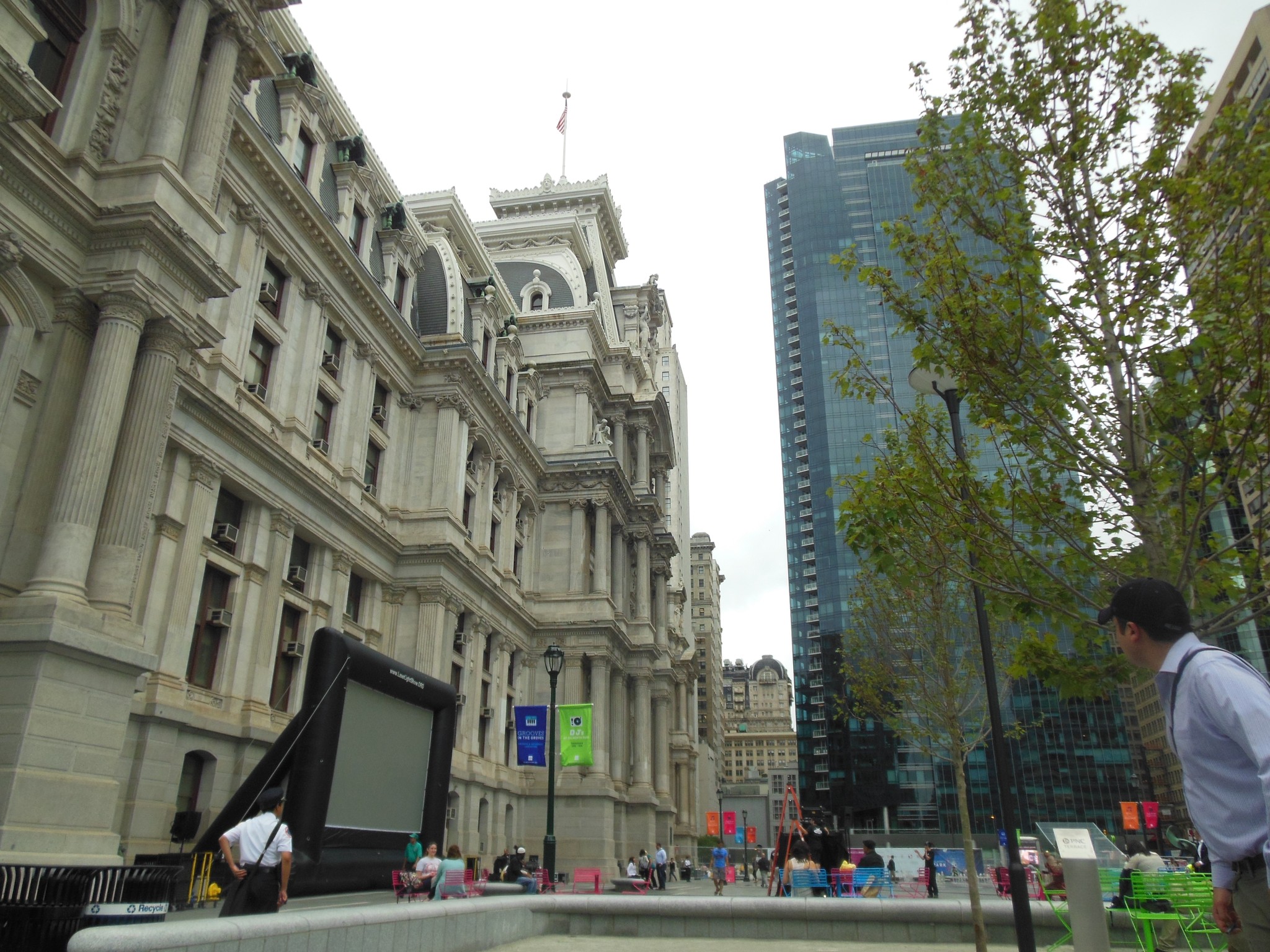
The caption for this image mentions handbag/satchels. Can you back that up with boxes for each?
[220,878,250,918]
[764,864,770,870]
[662,864,667,870]
[399,872,423,889]
[887,865,891,868]
[861,876,884,898]
[489,873,499,881]
[639,867,645,872]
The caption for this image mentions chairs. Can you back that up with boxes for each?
[391,870,431,904]
[779,868,792,897]
[935,864,1230,952]
[437,869,473,899]
[623,868,655,895]
[830,867,863,898]
[899,867,931,897]
[792,869,832,898]
[465,868,490,897]
[852,867,895,898]
[535,869,565,894]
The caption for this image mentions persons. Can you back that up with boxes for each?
[218,788,292,918]
[1095,577,1270,952]
[405,813,1212,911]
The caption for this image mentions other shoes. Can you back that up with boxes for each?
[928,894,938,898]
[761,885,768,888]
[753,878,758,883]
[660,888,665,890]
[656,887,660,890]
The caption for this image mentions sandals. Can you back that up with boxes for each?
[714,888,720,895]
[719,894,723,896]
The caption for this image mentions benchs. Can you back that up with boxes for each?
[572,867,606,895]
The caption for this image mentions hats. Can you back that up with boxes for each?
[253,787,283,810]
[1098,578,1189,633]
[863,840,875,849]
[517,847,525,853]
[409,833,418,839]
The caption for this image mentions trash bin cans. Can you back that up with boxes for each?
[680,867,686,880]
[0,864,100,952]
[79,865,182,930]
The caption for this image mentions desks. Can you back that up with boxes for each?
[824,873,852,897]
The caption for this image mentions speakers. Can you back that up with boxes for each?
[170,811,202,839]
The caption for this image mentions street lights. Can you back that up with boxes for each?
[907,356,1038,952]
[1130,774,1150,850]
[742,809,750,882]
[1152,804,1175,856]
[542,641,565,894]
[716,789,724,842]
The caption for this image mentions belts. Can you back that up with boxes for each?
[1233,855,1266,871]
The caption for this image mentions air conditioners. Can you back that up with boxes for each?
[465,528,472,541]
[453,633,467,646]
[310,439,330,456]
[457,693,467,706]
[281,641,305,659]
[211,522,240,544]
[372,405,387,421]
[364,484,377,500]
[258,281,279,303]
[243,382,268,403]
[466,460,477,474]
[205,608,234,629]
[286,565,307,584]
[478,841,484,853]
[493,491,502,503]
[479,707,494,719]
[446,807,456,820]
[515,518,523,529]
[505,720,516,731]
[321,353,341,372]
